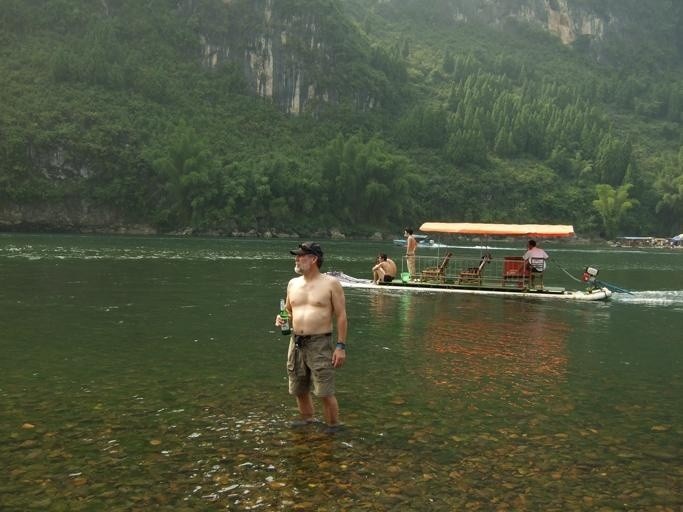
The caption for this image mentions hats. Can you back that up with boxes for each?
[290,241,321,256]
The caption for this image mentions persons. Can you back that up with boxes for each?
[371,253,392,285]
[377,253,396,280]
[515,239,549,274]
[404,228,417,280]
[274,241,348,435]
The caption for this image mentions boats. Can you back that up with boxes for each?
[317,223,613,304]
[391,232,434,248]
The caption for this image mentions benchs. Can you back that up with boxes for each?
[503,256,529,288]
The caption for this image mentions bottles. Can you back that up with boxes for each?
[278,298,292,336]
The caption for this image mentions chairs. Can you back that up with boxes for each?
[461,253,489,286]
[421,252,452,284]
[529,257,545,289]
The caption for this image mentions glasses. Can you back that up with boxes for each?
[298,243,320,256]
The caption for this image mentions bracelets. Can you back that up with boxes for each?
[336,342,346,350]
